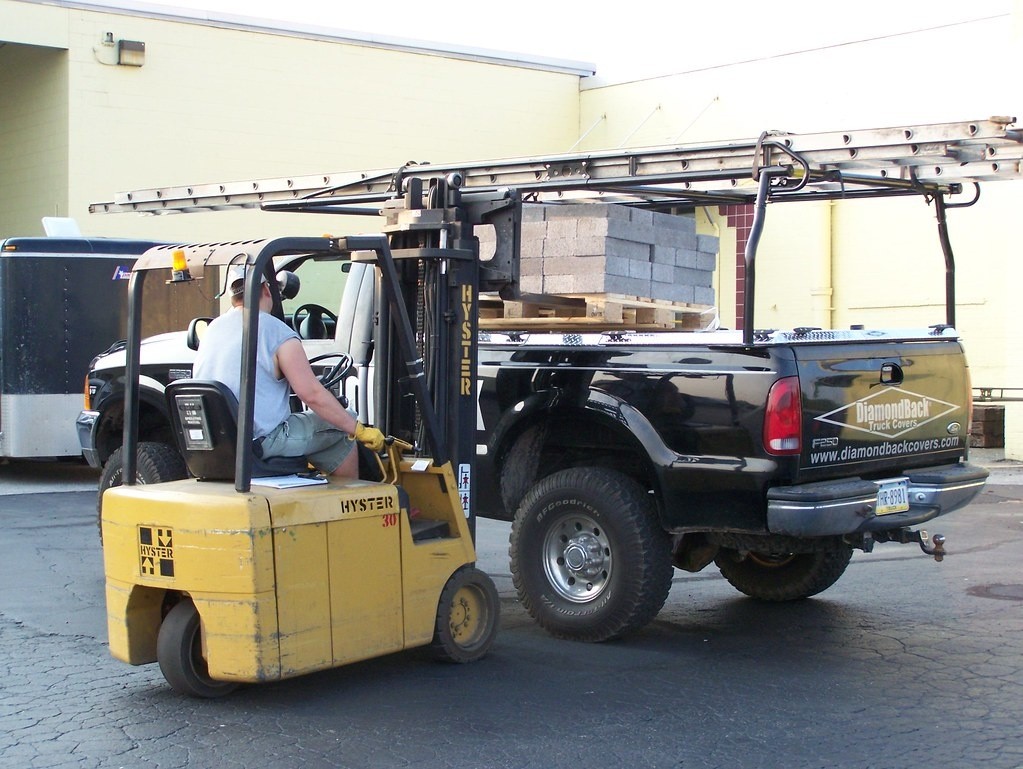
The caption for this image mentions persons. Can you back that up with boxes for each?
[192,261,386,482]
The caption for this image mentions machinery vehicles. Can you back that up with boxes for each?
[100,173,522,706]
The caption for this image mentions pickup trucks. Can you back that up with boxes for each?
[75,162,992,650]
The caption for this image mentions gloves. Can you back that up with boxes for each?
[349,421,385,453]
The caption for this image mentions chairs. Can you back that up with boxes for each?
[165,378,308,480]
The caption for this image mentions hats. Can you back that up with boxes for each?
[229,264,271,295]
